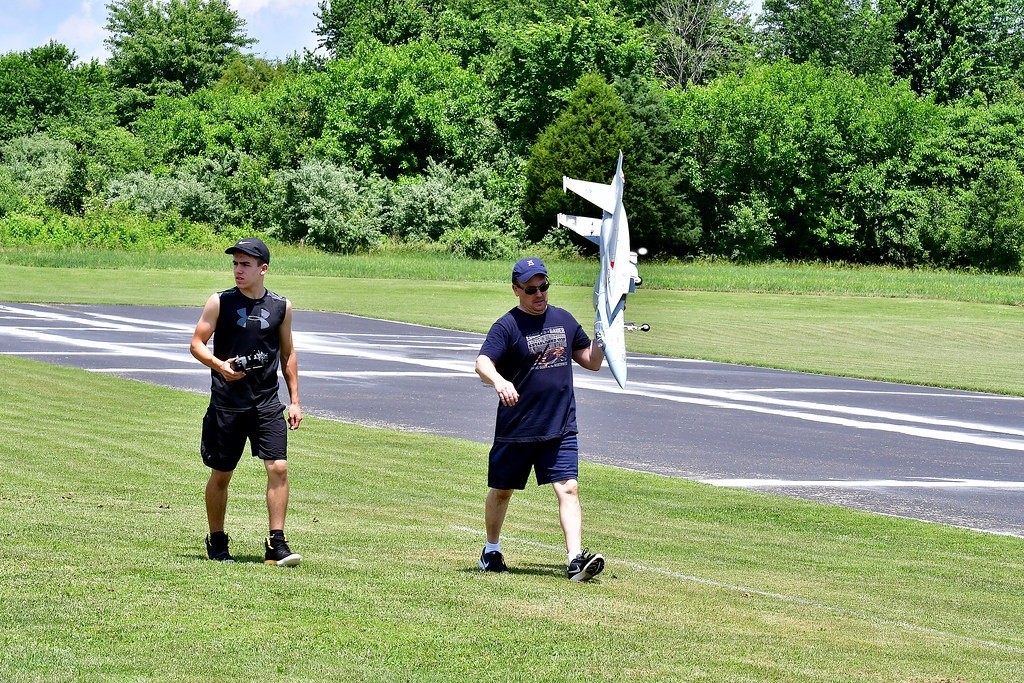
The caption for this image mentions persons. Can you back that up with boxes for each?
[190,237,304,567]
[474,257,606,582]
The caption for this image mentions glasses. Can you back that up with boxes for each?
[518,278,550,295]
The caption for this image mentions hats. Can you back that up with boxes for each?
[225,239,270,264]
[512,257,549,283]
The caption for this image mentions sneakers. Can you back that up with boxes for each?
[265,531,302,566]
[566,547,604,582]
[478,546,509,573]
[205,531,235,562]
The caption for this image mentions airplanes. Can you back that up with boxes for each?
[554,149,651,391]
